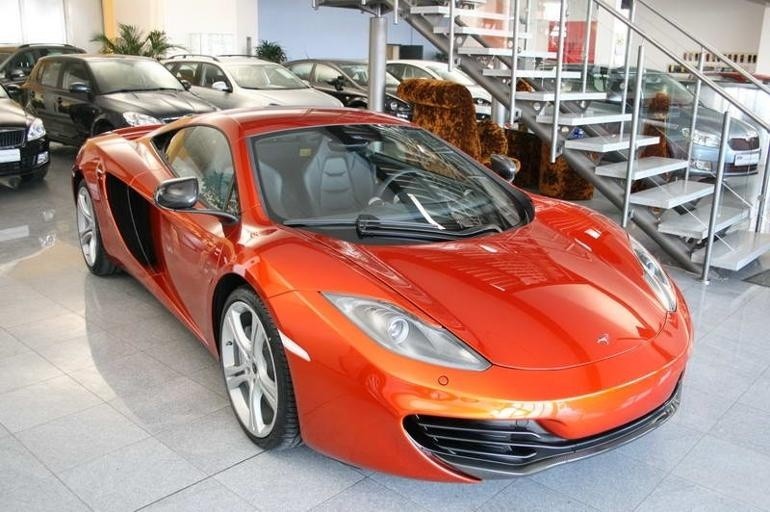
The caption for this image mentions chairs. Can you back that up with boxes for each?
[204,135,375,223]
[178,65,254,88]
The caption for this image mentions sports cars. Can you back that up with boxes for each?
[71,105,692,484]
